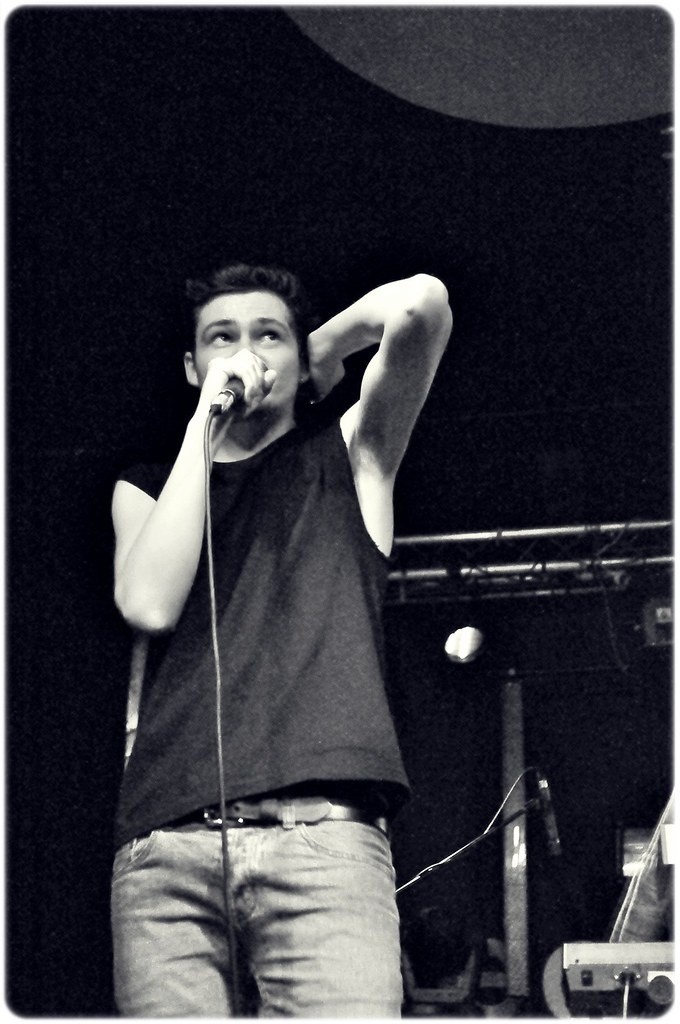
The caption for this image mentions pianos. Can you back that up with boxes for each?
[555,935,672,1018]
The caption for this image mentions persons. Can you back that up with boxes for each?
[109,262,456,1019]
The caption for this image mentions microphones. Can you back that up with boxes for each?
[211,378,244,416]
[535,771,563,856]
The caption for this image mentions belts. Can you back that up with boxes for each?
[156,796,372,830]
